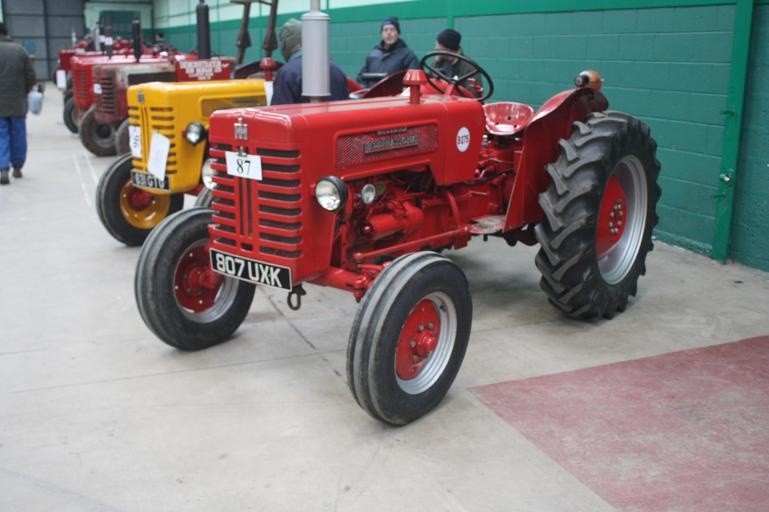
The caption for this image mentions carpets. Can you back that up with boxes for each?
[467,334,769,512]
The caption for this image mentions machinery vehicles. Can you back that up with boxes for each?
[51,0,285,162]
[131,3,661,429]
[93,74,389,249]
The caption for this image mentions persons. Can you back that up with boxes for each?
[85,20,177,59]
[270,16,348,105]
[356,17,420,88]
[0,21,37,185]
[430,29,484,103]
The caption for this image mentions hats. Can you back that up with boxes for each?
[278,18,303,54]
[436,29,461,50]
[380,17,400,36]
[1,168,23,185]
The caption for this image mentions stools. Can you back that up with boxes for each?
[482,101,534,145]
